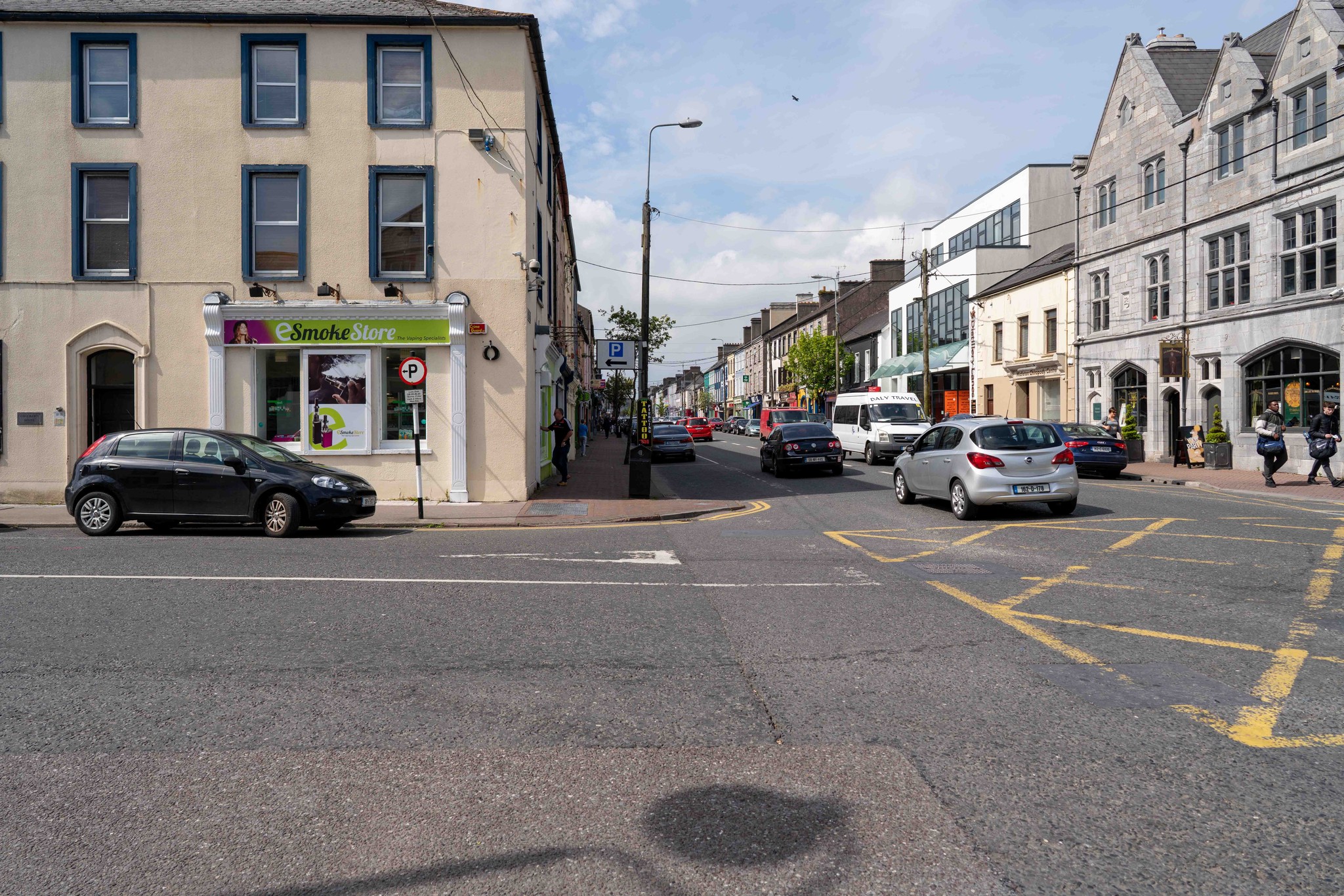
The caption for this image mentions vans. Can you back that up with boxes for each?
[760,407,810,447]
[832,392,936,466]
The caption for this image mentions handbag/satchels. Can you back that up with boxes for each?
[601,426,605,430]
[1257,434,1284,456]
[1307,437,1337,459]
[1099,416,1110,432]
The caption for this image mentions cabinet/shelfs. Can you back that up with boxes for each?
[386,393,426,440]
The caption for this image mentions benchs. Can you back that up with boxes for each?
[1065,427,1081,434]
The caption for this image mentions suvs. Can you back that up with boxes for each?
[721,416,745,433]
[934,413,1017,444]
[808,413,833,432]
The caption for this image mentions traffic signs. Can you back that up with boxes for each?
[399,357,427,386]
[596,339,635,370]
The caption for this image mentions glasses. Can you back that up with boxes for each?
[554,412,560,415]
[1111,412,1117,414]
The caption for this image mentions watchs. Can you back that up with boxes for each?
[562,440,565,442]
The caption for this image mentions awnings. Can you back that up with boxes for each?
[743,402,760,410]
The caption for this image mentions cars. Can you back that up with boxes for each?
[1012,421,1129,480]
[651,425,696,464]
[652,416,711,425]
[618,415,638,446]
[652,420,675,430]
[760,422,843,478]
[893,417,1079,521]
[733,419,749,435]
[745,419,760,437]
[709,417,725,431]
[683,416,713,442]
[64,428,377,538]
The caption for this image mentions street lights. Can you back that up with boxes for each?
[640,115,702,396]
[677,370,685,416]
[811,274,839,396]
[711,338,726,422]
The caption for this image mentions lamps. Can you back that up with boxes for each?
[249,282,276,300]
[573,368,581,377]
[384,282,403,301]
[550,325,590,345]
[317,282,340,300]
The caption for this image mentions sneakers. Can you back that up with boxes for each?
[557,481,568,486]
[581,453,587,457]
[1332,480,1344,487]
[1265,481,1276,487]
[559,475,571,483]
[1262,472,1275,484]
[1307,478,1319,485]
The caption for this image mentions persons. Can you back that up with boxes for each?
[540,407,574,486]
[866,407,885,421]
[1305,401,1344,487]
[578,417,588,457]
[308,355,367,405]
[1255,399,1288,487]
[229,320,258,344]
[1096,407,1125,442]
[941,412,951,422]
[773,413,782,421]
[598,412,619,439]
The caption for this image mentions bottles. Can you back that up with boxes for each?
[1303,431,1311,442]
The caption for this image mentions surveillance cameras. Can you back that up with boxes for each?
[513,251,522,257]
[535,278,546,286]
[529,261,540,273]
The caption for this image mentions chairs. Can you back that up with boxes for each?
[200,441,222,465]
[988,426,1018,446]
[183,439,201,463]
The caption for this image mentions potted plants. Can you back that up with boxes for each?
[1202,404,1233,470]
[1120,404,1143,463]
